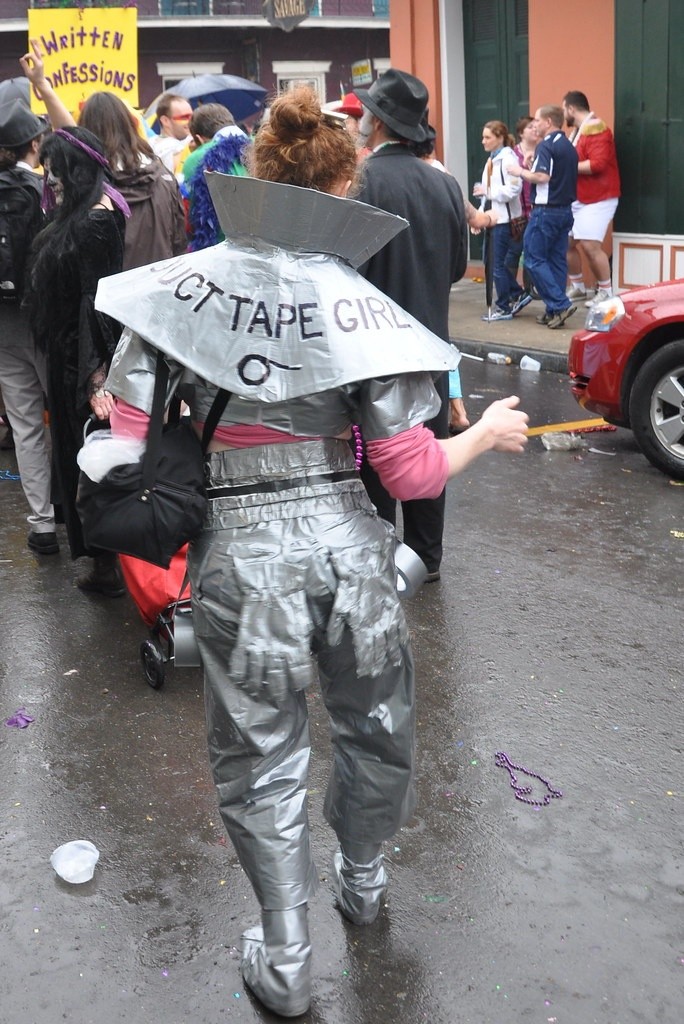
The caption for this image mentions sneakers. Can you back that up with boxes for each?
[482,308,513,321]
[510,290,533,314]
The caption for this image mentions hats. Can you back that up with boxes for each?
[0,76,30,105]
[354,69,429,142]
[0,97,49,149]
[331,92,361,117]
[417,108,437,142]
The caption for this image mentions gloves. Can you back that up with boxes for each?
[325,541,410,677]
[227,562,312,702]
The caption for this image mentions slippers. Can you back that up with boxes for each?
[449,419,468,433]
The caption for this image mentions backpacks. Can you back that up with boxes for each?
[0,164,44,304]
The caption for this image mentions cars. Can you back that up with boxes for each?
[568,279,684,481]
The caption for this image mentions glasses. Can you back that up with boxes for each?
[169,114,192,121]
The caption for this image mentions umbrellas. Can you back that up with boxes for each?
[143,70,269,135]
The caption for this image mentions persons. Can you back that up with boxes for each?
[346,68,468,581]
[471,91,622,328]
[103,88,530,1022]
[0,43,470,595]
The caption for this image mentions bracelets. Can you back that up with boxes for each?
[520,168,523,179]
[487,214,491,226]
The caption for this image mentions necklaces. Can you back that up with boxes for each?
[494,751,563,806]
[518,142,523,152]
[351,423,363,470]
[568,112,595,147]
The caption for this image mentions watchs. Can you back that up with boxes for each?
[96,390,103,397]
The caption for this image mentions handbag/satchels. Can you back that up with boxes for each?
[75,346,209,569]
[510,216,529,241]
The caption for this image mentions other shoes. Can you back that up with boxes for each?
[77,555,127,596]
[547,304,578,328]
[527,289,541,300]
[537,312,564,327]
[27,528,60,556]
[584,286,610,307]
[565,285,586,302]
[424,567,440,584]
